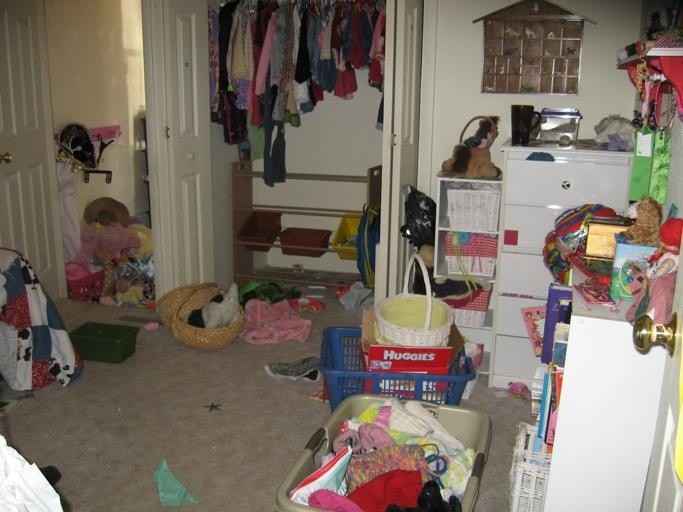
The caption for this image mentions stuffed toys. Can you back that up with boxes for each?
[625,215,683,328]
[619,197,663,246]
[457,338,481,370]
[398,185,483,298]
[440,143,501,178]
[593,114,637,149]
[180,281,241,330]
[462,114,499,151]
[385,478,462,512]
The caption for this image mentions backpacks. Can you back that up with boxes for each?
[358,207,379,288]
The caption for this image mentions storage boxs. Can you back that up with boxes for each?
[444,233,499,278]
[446,189,503,231]
[435,277,493,328]
[272,391,492,511]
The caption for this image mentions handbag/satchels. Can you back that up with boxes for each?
[401,185,435,249]
[442,116,501,178]
[345,443,448,512]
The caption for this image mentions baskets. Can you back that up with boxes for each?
[158,281,245,350]
[316,327,476,409]
[65,261,105,302]
[509,421,549,512]
[378,257,454,346]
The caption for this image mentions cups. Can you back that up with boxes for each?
[510,104,541,147]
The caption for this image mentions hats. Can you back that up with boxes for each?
[84,197,130,229]
[125,224,152,256]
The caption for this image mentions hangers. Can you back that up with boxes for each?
[210,2,385,29]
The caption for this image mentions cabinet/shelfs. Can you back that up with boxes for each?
[486,141,634,391]
[508,257,669,512]
[431,175,504,353]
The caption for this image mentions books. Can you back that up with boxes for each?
[361,344,454,405]
[519,283,574,471]
[573,282,615,305]
